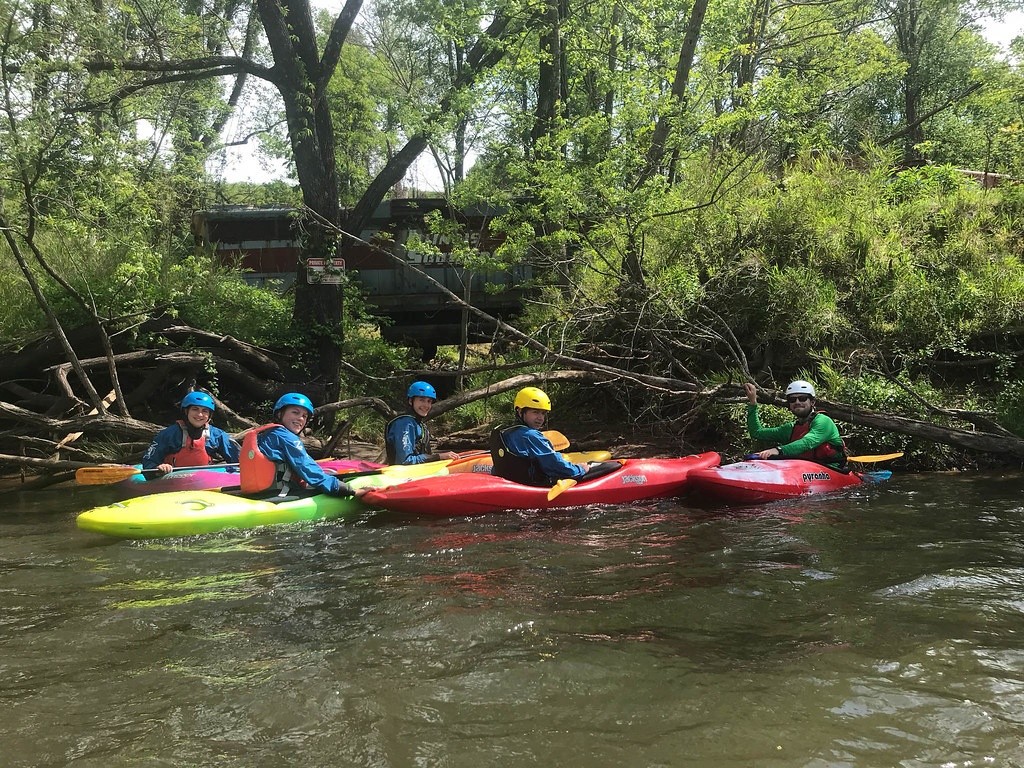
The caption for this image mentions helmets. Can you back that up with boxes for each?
[273,393,314,420]
[514,387,551,411]
[407,381,437,400]
[786,381,815,397]
[180,392,215,423]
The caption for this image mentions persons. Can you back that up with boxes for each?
[141,392,239,474]
[240,392,379,499]
[368,233,379,245]
[489,387,602,482]
[384,381,460,466]
[742,380,848,470]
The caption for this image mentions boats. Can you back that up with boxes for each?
[363,451,721,515]
[120,458,387,493]
[75,473,407,538]
[375,448,611,482]
[685,459,862,502]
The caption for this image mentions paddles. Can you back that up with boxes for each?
[75,430,576,502]
[744,453,905,462]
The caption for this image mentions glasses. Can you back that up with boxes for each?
[788,396,812,403]
